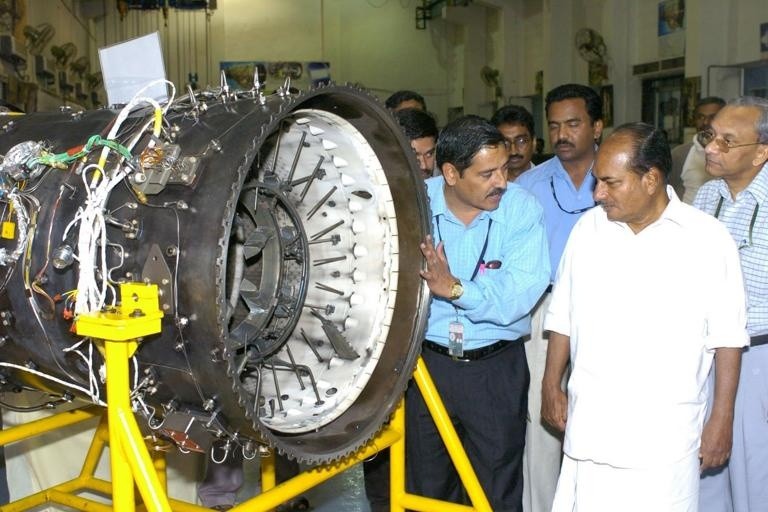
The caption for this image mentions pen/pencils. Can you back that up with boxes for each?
[479,260,486,275]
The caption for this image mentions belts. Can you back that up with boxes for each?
[422,338,508,361]
[750,336,767,345]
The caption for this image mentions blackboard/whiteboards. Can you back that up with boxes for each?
[98,31,169,107]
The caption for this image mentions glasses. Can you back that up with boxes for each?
[504,138,531,150]
[703,130,758,153]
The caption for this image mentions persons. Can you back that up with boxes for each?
[659,1,684,36]
[195,434,315,511]
[361,84,767,512]
